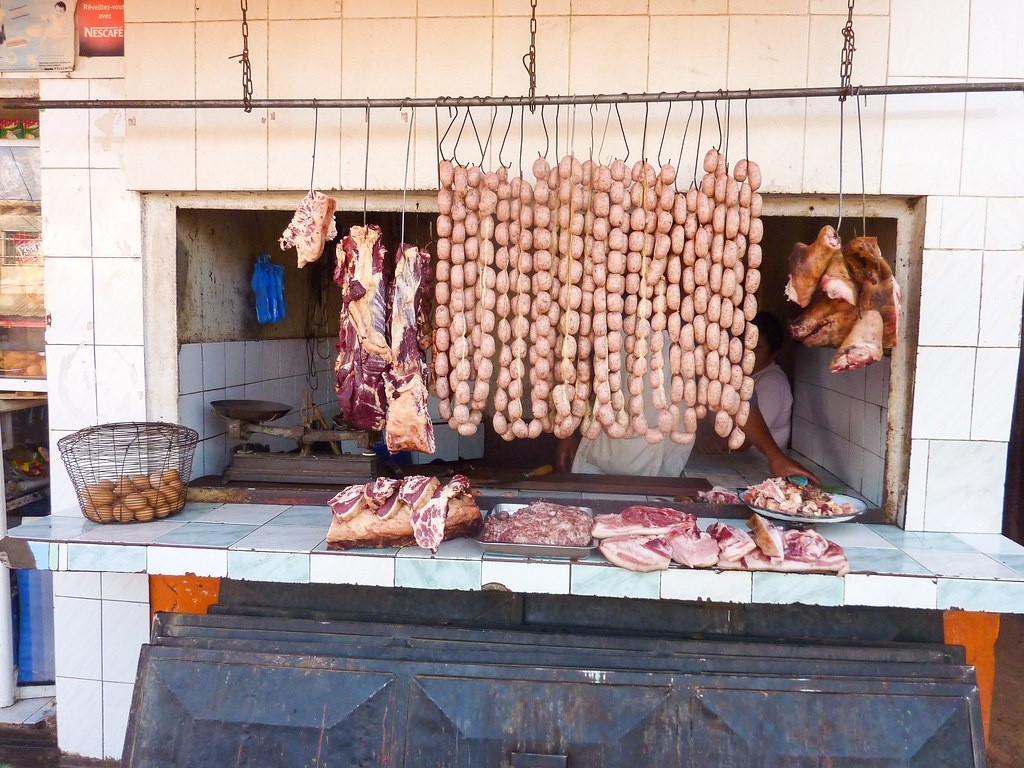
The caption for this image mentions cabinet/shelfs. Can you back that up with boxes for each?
[0,391,50,537]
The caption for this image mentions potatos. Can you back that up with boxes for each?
[82,469,185,523]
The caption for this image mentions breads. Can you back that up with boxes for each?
[0,349,46,376]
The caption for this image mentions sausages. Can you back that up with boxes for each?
[434,149,763,451]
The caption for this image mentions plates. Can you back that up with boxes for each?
[739,489,869,522]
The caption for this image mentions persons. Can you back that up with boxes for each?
[556,282,820,484]
